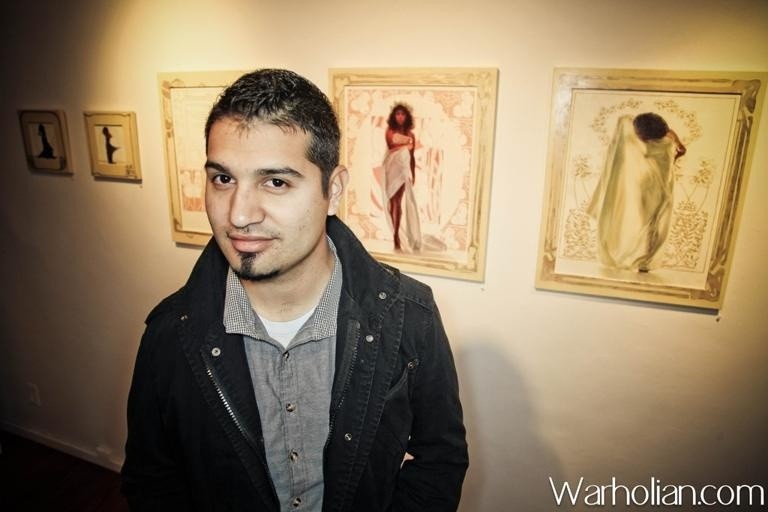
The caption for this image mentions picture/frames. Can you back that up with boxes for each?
[83,110,142,180]
[17,109,74,176]
[327,67,499,283]
[157,69,249,246]
[534,67,768,306]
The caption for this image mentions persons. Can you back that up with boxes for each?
[111,70,470,511]
[374,100,422,250]
